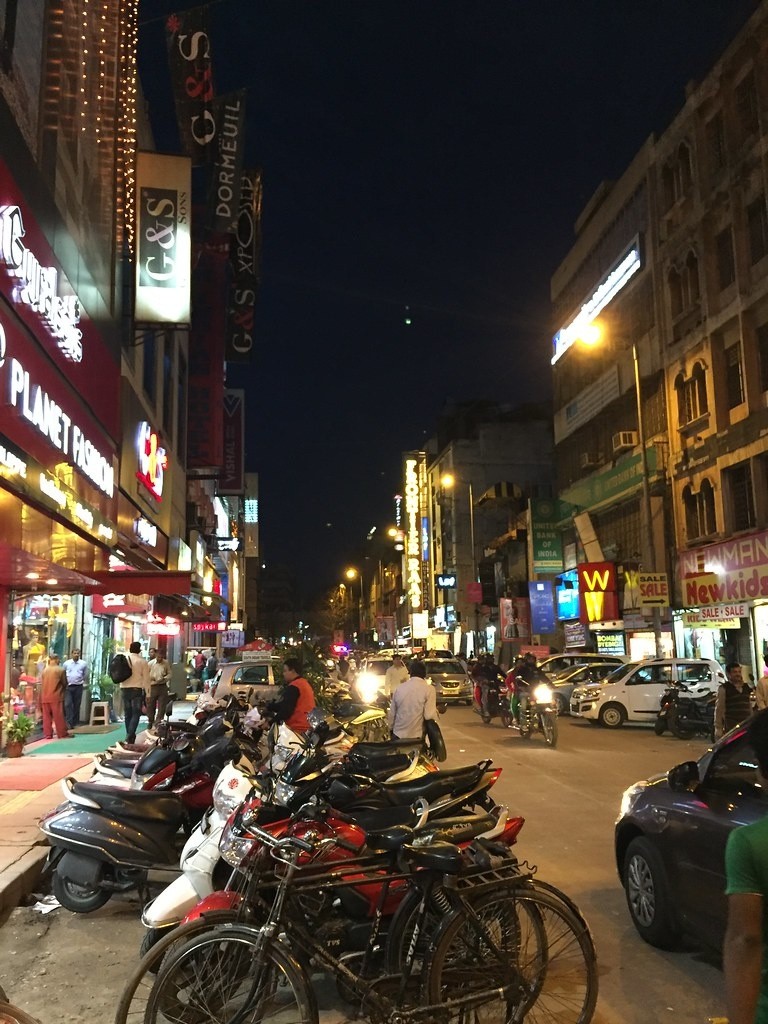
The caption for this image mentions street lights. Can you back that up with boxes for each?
[347,569,365,649]
[443,473,479,655]
[389,527,414,654]
[579,321,663,657]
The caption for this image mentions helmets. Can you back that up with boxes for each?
[523,651,537,665]
[513,655,524,665]
[485,652,495,664]
[477,653,484,660]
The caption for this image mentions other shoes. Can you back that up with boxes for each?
[512,720,519,726]
[147,722,152,730]
[521,724,529,731]
[484,710,490,716]
[478,705,482,712]
[63,733,74,738]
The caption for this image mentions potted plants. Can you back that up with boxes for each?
[4,709,34,758]
[84,630,124,726]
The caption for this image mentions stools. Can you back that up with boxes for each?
[88,702,110,726]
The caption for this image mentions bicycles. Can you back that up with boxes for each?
[116,771,599,1024]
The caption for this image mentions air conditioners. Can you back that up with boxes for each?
[612,431,640,455]
[580,453,596,469]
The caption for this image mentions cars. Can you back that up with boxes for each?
[532,653,625,717]
[615,706,768,945]
[322,659,336,670]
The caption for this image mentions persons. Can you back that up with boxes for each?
[22,629,46,678]
[149,648,156,661]
[257,658,316,779]
[471,653,559,731]
[385,655,410,699]
[388,662,446,742]
[63,649,87,730]
[193,649,207,679]
[755,654,768,710]
[120,642,151,744]
[714,663,756,742]
[336,656,349,677]
[42,653,69,739]
[206,652,217,679]
[723,707,768,1024]
[147,650,173,730]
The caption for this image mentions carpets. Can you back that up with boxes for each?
[69,723,119,735]
[28,720,148,755]
[0,758,96,792]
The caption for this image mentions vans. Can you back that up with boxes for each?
[208,660,285,704]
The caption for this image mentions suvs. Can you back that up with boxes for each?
[355,656,475,705]
[569,658,728,728]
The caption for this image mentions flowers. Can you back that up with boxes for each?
[0,688,27,720]
[19,675,40,687]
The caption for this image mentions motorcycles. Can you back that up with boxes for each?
[473,676,513,727]
[272,702,525,931]
[654,672,726,738]
[508,674,558,748]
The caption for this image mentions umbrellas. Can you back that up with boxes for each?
[0,538,102,749]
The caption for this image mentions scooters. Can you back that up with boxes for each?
[32,686,278,974]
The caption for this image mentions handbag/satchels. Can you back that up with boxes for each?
[109,654,132,685]
